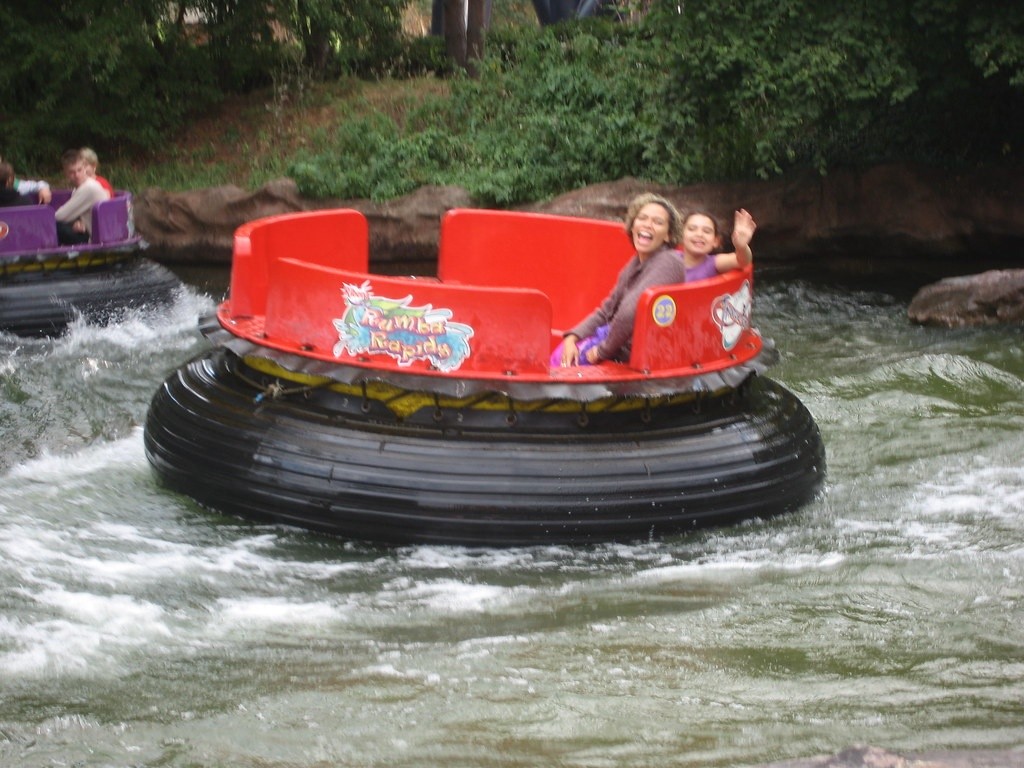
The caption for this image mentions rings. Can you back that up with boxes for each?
[562,361,566,363]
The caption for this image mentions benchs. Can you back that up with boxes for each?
[0,188,144,274]
[215,205,765,395]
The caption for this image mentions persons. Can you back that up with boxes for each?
[80,148,114,197]
[675,207,756,281]
[53,150,111,244]
[551,193,685,372]
[14,179,51,204]
[0,162,29,206]
